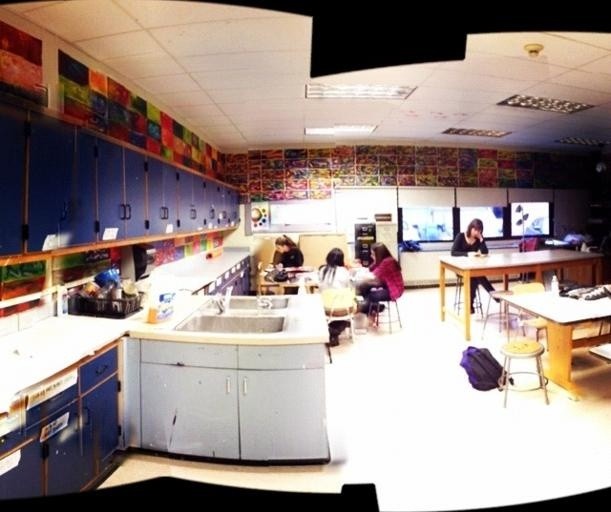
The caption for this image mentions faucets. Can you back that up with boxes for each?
[224,286,234,308]
[211,297,226,312]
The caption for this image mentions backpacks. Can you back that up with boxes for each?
[460,346,514,391]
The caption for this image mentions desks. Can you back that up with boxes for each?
[266,267,316,294]
[440,249,603,342]
[505,288,611,399]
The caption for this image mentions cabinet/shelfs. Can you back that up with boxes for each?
[178,164,205,238]
[230,188,240,225]
[23,341,122,496]
[219,183,230,230]
[146,152,179,248]
[141,340,330,466]
[25,109,98,257]
[97,130,148,250]
[194,254,251,295]
[205,177,219,236]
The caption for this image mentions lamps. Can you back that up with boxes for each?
[306,125,376,136]
[556,136,608,148]
[498,94,592,116]
[442,128,510,140]
[306,86,413,100]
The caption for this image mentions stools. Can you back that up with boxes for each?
[454,274,484,319]
[484,291,526,337]
[498,339,549,407]
[367,298,402,335]
[327,315,355,346]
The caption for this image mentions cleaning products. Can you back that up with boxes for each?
[57,282,68,318]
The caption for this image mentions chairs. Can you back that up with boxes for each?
[514,282,549,344]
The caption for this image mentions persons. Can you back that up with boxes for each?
[451,219,501,315]
[357,243,404,315]
[318,248,355,347]
[273,234,304,268]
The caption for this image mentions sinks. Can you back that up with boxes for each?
[207,299,289,308]
[180,315,284,333]
